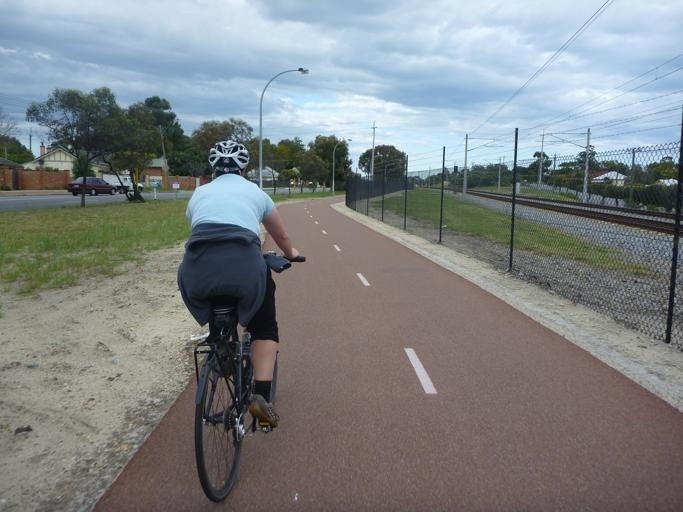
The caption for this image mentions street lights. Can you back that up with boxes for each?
[332,138,353,192]
[259,67,310,189]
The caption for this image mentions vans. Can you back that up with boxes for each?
[102,173,144,194]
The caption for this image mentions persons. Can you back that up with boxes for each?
[177,140,299,427]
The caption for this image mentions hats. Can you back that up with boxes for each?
[208,140,249,172]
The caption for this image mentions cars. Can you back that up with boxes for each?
[68,176,116,196]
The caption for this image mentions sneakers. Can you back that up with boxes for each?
[248,395,280,428]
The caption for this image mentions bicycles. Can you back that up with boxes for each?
[194,250,306,505]
